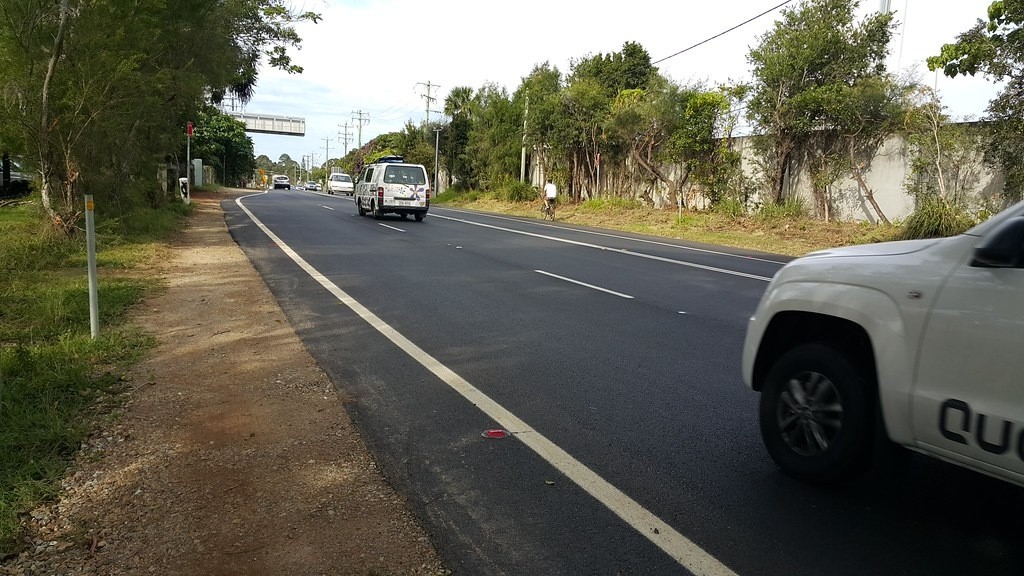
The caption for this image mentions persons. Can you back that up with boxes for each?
[544,179,557,211]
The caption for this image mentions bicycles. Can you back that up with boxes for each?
[539,194,555,221]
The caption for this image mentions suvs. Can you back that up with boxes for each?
[274,175,290,190]
[305,181,316,191]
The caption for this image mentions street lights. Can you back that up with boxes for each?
[432,127,444,197]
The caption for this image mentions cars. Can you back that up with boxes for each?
[294,185,303,191]
[290,185,293,190]
[0,159,33,191]
[740,196,1023,526]
[316,184,321,191]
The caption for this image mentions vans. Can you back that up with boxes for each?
[327,173,354,196]
[353,160,430,223]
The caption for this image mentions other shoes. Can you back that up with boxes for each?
[545,206,549,210]
